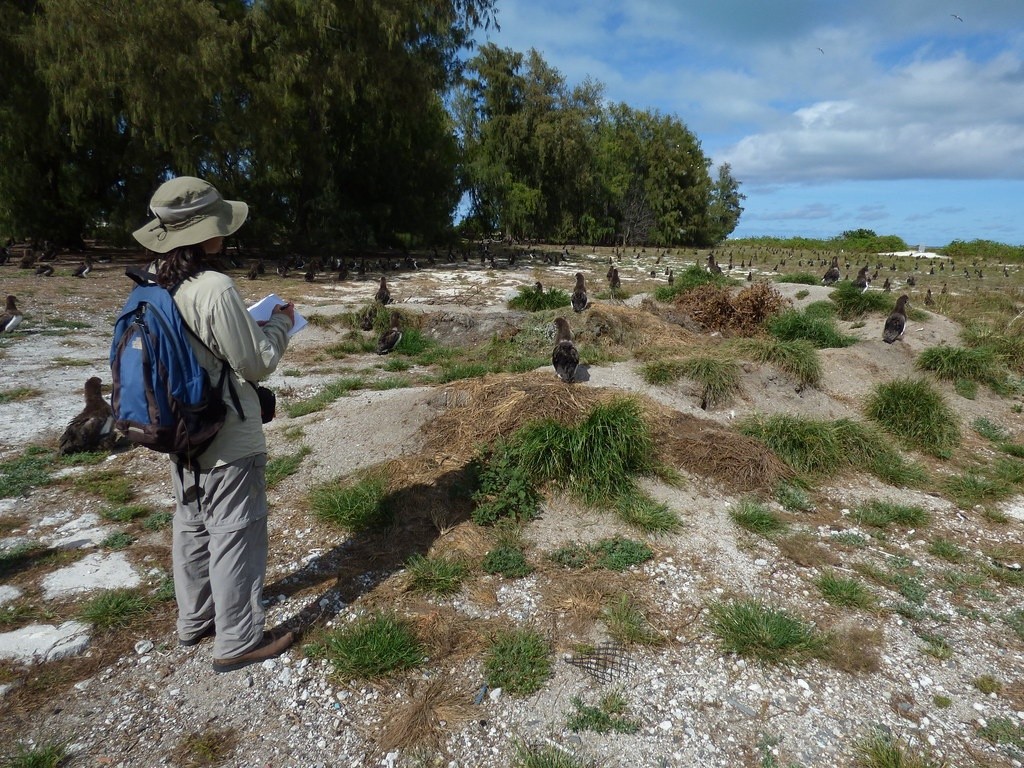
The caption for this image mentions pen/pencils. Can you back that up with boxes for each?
[280,302,291,310]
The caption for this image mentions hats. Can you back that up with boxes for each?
[131,176,248,254]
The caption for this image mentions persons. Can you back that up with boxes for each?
[136,176,296,673]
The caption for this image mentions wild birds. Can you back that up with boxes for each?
[0,233,1024,456]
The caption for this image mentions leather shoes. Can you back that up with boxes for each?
[213,630,296,672]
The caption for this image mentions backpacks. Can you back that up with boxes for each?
[109,265,233,461]
